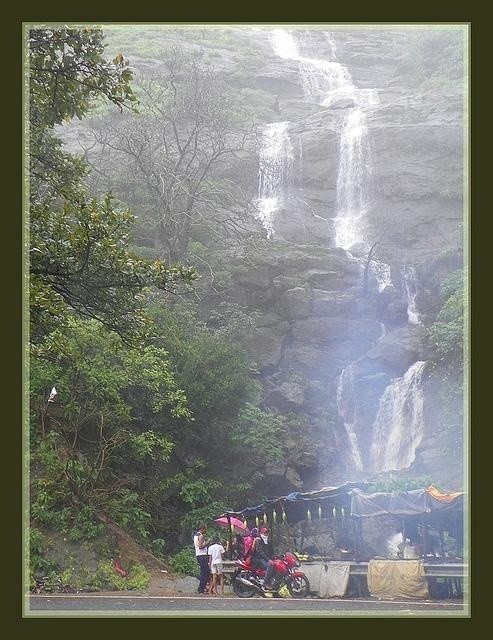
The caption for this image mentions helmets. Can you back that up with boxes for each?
[250,527,259,536]
[261,527,269,535]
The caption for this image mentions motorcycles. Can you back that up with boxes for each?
[228,552,310,599]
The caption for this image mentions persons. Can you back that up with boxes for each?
[194,524,284,597]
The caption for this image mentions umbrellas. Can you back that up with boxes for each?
[214,515,246,530]
